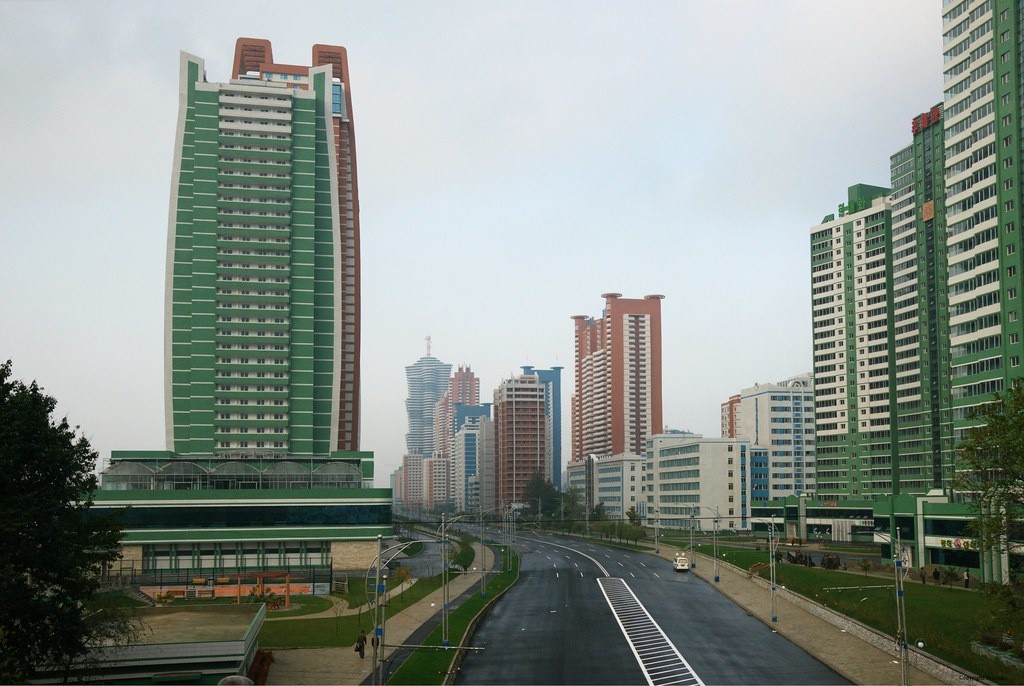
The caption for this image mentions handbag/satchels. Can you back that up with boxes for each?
[355,644,359,651]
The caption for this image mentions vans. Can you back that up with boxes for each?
[672,557,689,572]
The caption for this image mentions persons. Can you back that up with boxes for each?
[919,567,926,584]
[786,549,812,568]
[933,568,940,585]
[775,549,783,563]
[820,552,841,570]
[372,634,379,657]
[963,569,970,588]
[356,630,367,658]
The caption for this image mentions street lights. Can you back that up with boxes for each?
[553,498,566,534]
[745,518,781,622]
[696,506,723,583]
[364,539,445,686]
[436,514,479,649]
[675,503,696,568]
[641,501,660,554]
[528,498,543,536]
[476,507,509,599]
[849,530,911,686]
